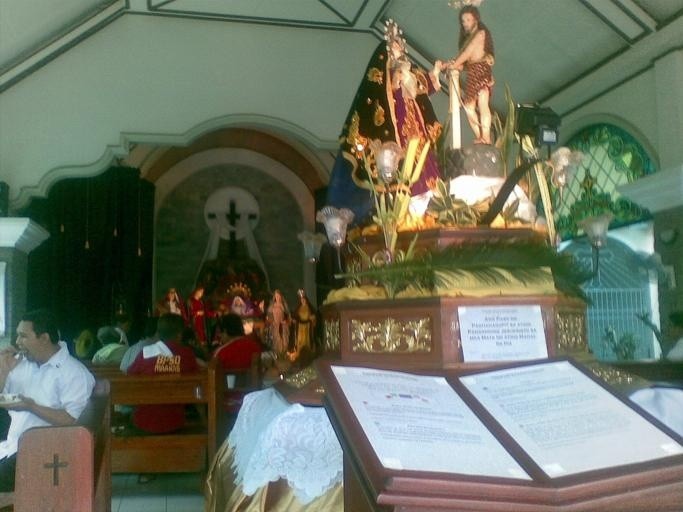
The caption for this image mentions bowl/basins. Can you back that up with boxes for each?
[261,376,281,386]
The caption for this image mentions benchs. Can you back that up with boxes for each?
[0,345,259,512]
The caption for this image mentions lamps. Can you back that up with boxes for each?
[545,147,585,214]
[316,207,354,274]
[372,141,406,196]
[576,209,613,273]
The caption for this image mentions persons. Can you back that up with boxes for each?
[68,306,268,483]
[291,296,317,355]
[164,290,181,314]
[266,289,288,354]
[445,5,497,146]
[231,296,253,318]
[0,308,96,494]
[383,39,443,149]
[185,288,206,345]
[663,310,682,361]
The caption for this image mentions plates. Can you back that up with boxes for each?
[0,398,21,405]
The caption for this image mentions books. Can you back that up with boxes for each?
[326,356,683,473]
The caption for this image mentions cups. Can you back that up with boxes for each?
[226,375,236,389]
[0,393,18,401]
